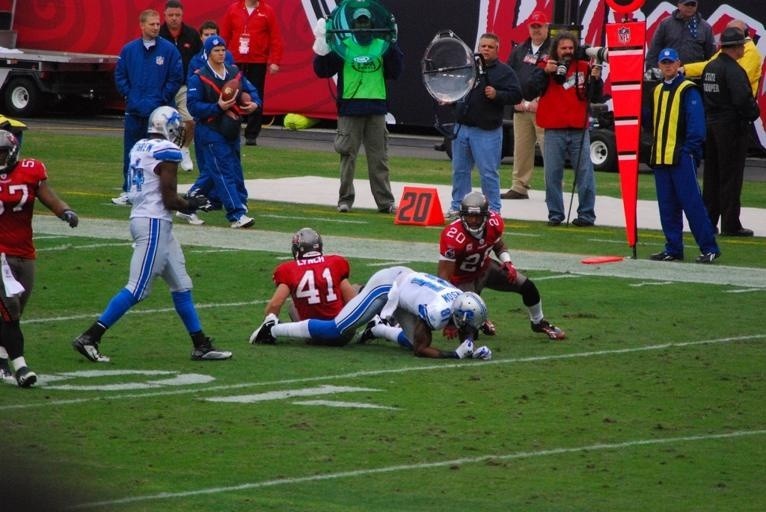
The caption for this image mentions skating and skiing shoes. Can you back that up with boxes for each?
[337,201,351,212]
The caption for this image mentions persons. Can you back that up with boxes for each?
[312,0,407,212]
[641,1,765,262]
[438,191,567,340]
[438,11,604,227]
[264,226,362,320]
[114,0,280,229]
[0,129,79,388]
[248,265,492,360]
[72,105,232,362]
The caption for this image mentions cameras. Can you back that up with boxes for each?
[556,56,568,81]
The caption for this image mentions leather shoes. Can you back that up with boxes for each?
[720,225,754,237]
[245,136,257,146]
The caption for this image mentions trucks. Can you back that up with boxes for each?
[0,29,120,121]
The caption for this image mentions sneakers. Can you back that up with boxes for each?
[174,208,206,226]
[229,213,256,229]
[548,214,592,228]
[695,250,723,263]
[481,319,496,336]
[0,365,19,387]
[15,365,37,386]
[178,147,194,172]
[186,187,213,211]
[500,188,530,199]
[248,312,281,343]
[356,314,384,344]
[110,193,131,206]
[72,332,110,363]
[530,317,564,340]
[649,249,684,261]
[190,337,233,361]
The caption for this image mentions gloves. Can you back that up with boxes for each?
[442,324,459,341]
[57,207,79,228]
[503,260,518,286]
[455,338,475,359]
[472,345,493,361]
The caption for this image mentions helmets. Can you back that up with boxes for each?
[145,104,187,151]
[716,26,752,48]
[459,191,492,218]
[451,291,488,330]
[0,130,23,175]
[291,226,324,261]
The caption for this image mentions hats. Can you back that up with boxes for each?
[679,0,699,6]
[525,11,548,29]
[203,34,226,59]
[656,47,679,65]
[352,6,373,21]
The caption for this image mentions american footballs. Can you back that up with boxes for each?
[222,79,239,100]
[237,92,251,107]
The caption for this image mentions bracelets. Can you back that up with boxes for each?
[439,351,459,358]
